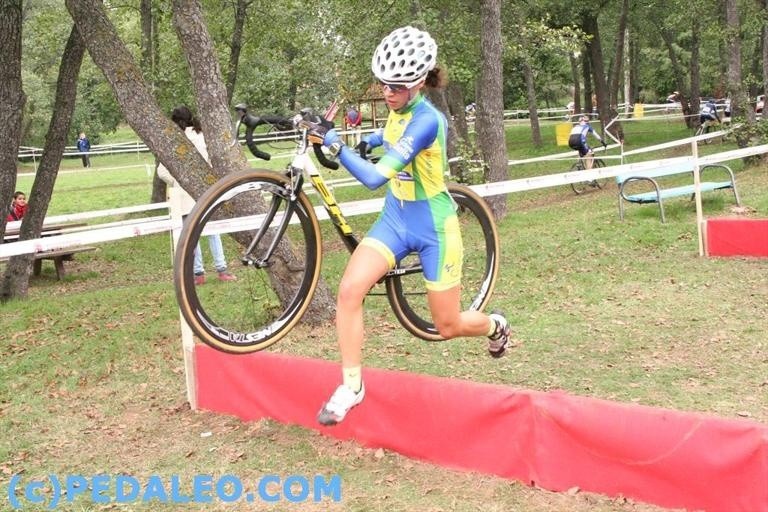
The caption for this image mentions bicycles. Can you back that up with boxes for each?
[661,102,688,123]
[700,121,731,145]
[172,102,502,356]
[568,145,607,195]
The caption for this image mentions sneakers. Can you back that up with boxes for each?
[315,377,368,427]
[194,273,207,285]
[217,268,238,282]
[486,311,511,359]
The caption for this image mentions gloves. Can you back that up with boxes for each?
[353,138,372,162]
[296,110,335,147]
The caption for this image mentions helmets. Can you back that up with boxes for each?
[368,25,440,90]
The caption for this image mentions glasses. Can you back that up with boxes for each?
[374,73,430,94]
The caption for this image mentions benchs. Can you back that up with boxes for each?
[0,223,97,279]
[618,164,740,222]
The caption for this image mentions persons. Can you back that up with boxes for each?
[666,91,679,113]
[78,132,92,167]
[157,104,238,282]
[700,98,721,133]
[346,106,360,146]
[565,102,574,119]
[724,94,732,117]
[465,102,476,116]
[592,93,599,119]
[301,26,510,425]
[568,116,607,186]
[7,191,29,223]
